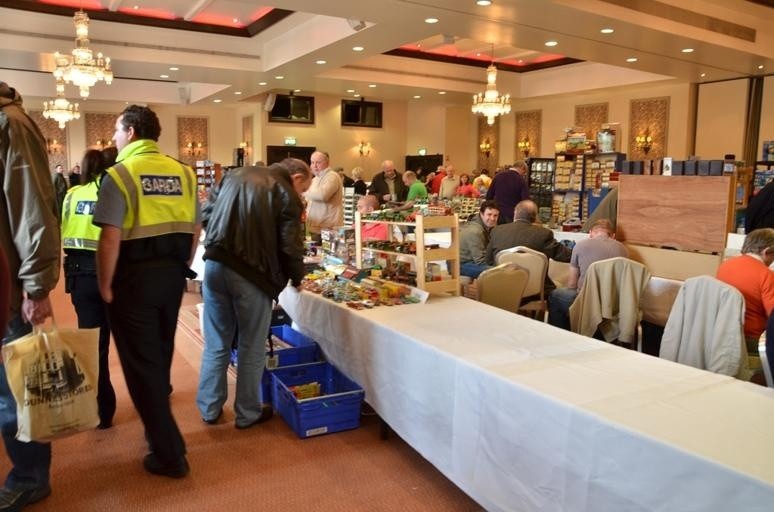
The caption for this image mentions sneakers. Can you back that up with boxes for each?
[96,415,113,429]
[235,405,272,429]
[202,406,223,423]
[0,477,51,510]
[143,452,190,478]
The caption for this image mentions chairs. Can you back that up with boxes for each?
[660,275,762,380]
[568,257,650,354]
[495,245,549,320]
[476,260,529,314]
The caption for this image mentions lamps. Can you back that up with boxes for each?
[357,141,372,159]
[468,41,515,127]
[51,5,116,90]
[479,137,494,157]
[184,140,203,158]
[42,78,80,131]
[636,126,654,155]
[517,135,532,155]
[45,138,57,155]
[94,136,112,149]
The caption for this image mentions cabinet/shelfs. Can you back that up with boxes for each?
[525,158,555,221]
[617,172,737,255]
[750,161,774,199]
[192,162,220,193]
[552,152,627,226]
[353,210,464,299]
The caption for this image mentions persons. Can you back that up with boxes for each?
[546,218,630,332]
[715,228,773,388]
[54,146,162,429]
[298,150,342,243]
[745,177,772,383]
[0,78,62,510]
[484,161,528,218]
[446,199,499,279]
[196,158,310,434]
[485,199,574,307]
[90,105,200,477]
[334,161,495,211]
[580,186,617,234]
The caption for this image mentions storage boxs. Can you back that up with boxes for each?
[196,299,367,438]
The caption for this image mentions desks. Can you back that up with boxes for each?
[543,229,773,330]
[278,250,769,510]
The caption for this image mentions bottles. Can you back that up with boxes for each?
[414,195,429,205]
[722,154,735,177]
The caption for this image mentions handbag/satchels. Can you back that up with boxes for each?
[2,314,106,443]
